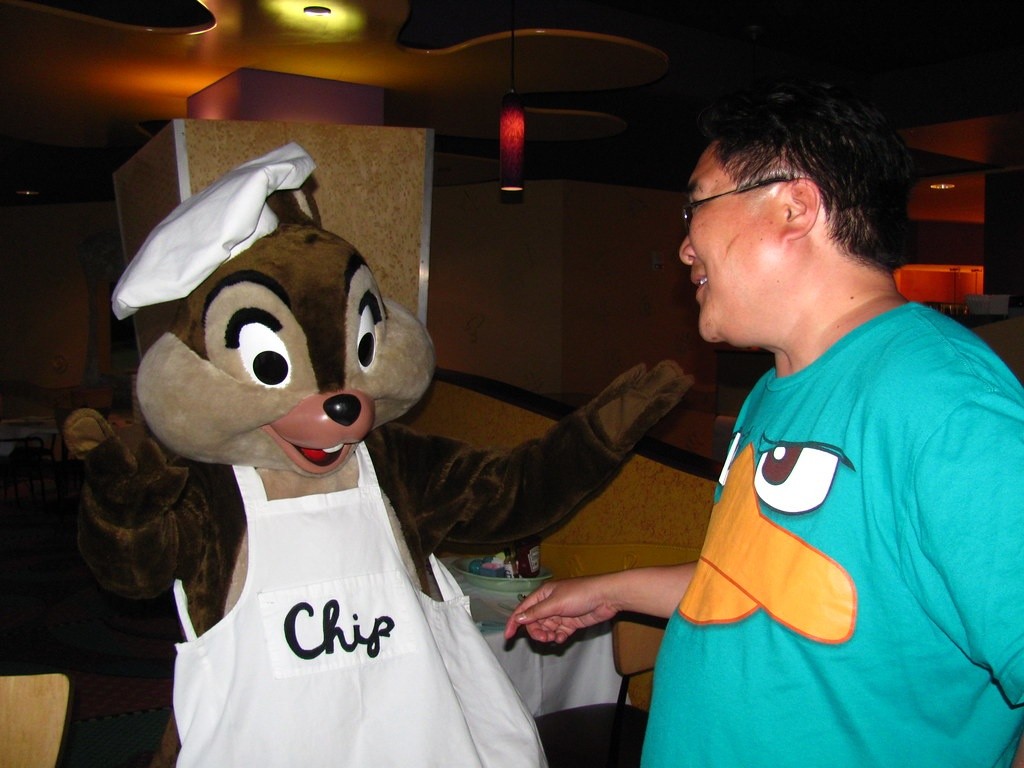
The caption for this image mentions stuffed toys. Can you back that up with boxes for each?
[53,143,696,767]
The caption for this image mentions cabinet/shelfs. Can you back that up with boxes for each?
[951,306,1024,386]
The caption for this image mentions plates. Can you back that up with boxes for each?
[450,556,553,592]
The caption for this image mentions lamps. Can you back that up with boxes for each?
[497,31,524,194]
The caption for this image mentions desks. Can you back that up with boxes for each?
[1,412,127,460]
[438,553,631,716]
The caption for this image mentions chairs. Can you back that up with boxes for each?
[606,616,667,765]
[1,436,67,507]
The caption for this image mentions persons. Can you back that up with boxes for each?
[506,90,1023,766]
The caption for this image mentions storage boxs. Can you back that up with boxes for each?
[967,292,1009,317]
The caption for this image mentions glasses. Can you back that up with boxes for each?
[678,174,794,225]
